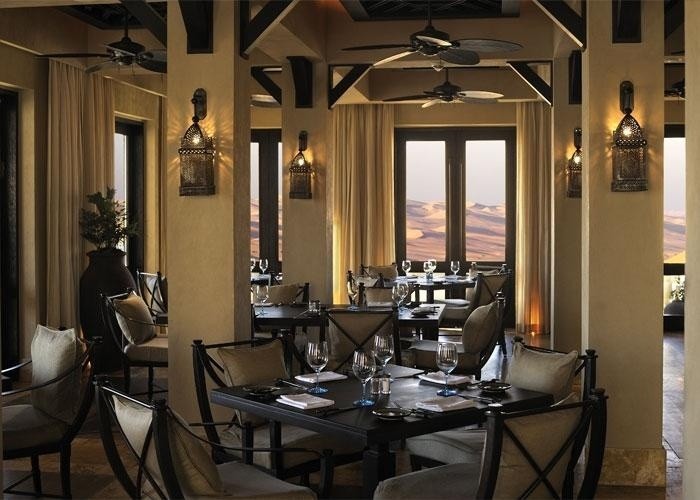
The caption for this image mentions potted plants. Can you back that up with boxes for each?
[80,186,138,374]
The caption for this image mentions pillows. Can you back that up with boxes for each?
[472,274,508,305]
[328,314,393,359]
[31,324,83,426]
[369,266,397,281]
[476,392,586,500]
[462,301,498,355]
[111,394,234,498]
[253,283,299,306]
[466,269,498,301]
[113,290,156,345]
[365,289,392,305]
[147,277,168,315]
[217,338,291,427]
[504,341,578,403]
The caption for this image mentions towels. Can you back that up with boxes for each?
[276,393,335,410]
[416,396,476,412]
[294,371,348,383]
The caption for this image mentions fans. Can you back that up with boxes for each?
[34,10,167,74]
[341,0,522,65]
[382,68,504,108]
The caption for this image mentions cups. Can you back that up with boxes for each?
[451,261,460,279]
[436,343,458,397]
[309,298,320,320]
[306,341,328,394]
[424,260,436,284]
[352,351,376,406]
[374,334,393,375]
[256,286,268,316]
[250,259,255,272]
[347,282,359,311]
[392,282,408,315]
[259,259,268,278]
[402,261,411,281]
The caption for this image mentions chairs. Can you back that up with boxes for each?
[91,374,319,500]
[251,279,310,373]
[358,282,420,305]
[96,287,168,402]
[372,387,609,500]
[345,270,384,305]
[191,328,363,486]
[320,305,402,367]
[434,269,513,354]
[473,263,505,271]
[405,335,598,473]
[359,262,398,276]
[408,291,506,381]
[1,326,102,500]
[137,272,168,326]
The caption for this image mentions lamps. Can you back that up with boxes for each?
[289,130,312,199]
[566,127,582,198]
[610,80,649,191]
[177,88,216,197]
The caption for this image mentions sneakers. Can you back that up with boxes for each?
[417,371,470,383]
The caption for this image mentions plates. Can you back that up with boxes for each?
[477,382,512,393]
[411,311,429,318]
[369,408,415,422]
[243,385,280,396]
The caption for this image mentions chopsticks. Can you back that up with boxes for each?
[275,378,301,388]
[458,394,495,404]
[317,407,361,419]
